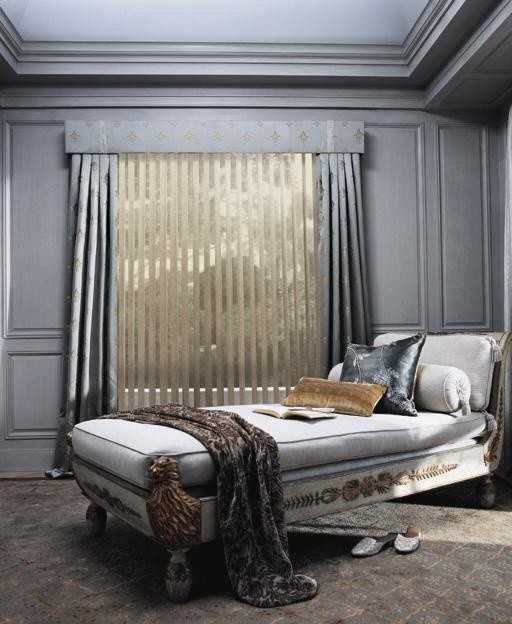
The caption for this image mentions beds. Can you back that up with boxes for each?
[67,331,512,602]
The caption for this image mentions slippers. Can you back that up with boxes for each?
[351,533,397,556]
[394,527,420,552]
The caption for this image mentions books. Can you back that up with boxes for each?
[252,406,337,420]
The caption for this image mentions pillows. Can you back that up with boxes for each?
[281,333,502,431]
